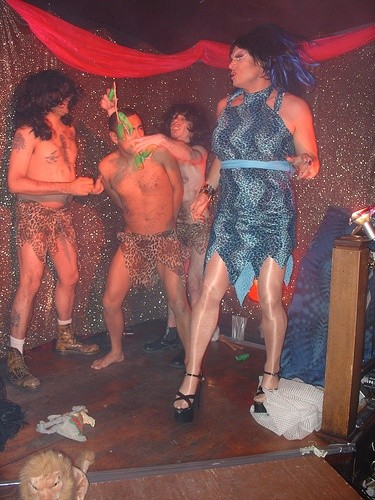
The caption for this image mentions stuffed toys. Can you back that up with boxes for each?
[19,448,95,500]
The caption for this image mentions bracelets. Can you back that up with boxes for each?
[198,184,215,199]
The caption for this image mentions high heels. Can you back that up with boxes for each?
[254,368,283,413]
[173,371,203,422]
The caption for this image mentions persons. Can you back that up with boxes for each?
[99,88,211,367]
[91,108,192,369]
[6,69,104,393]
[174,23,319,422]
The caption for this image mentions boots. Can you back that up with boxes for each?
[54,323,101,355]
[7,346,40,391]
[142,327,178,352]
